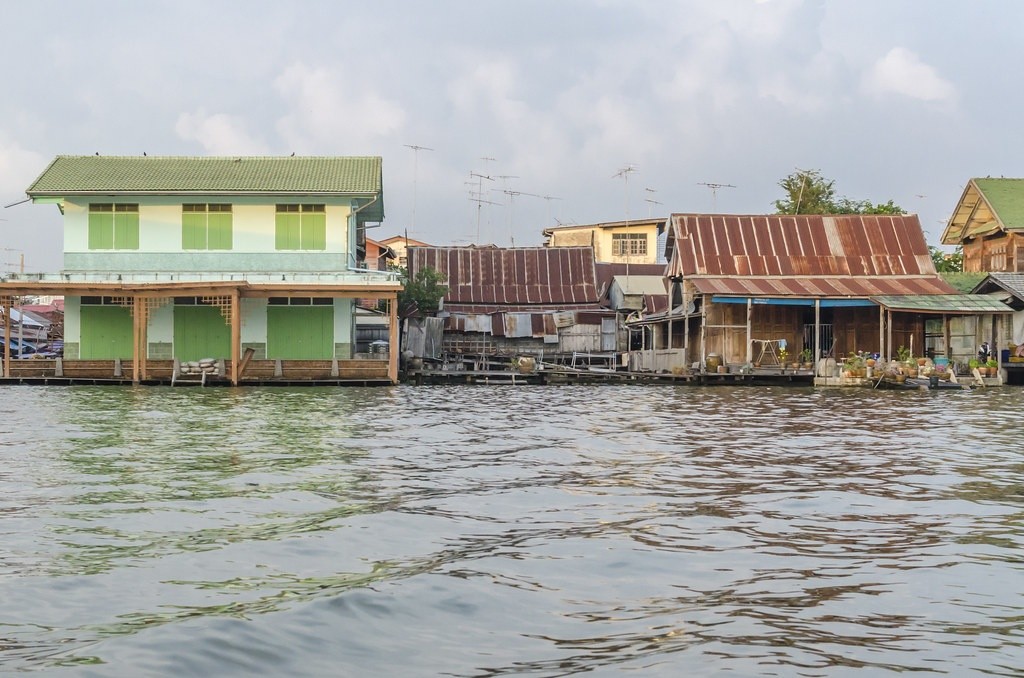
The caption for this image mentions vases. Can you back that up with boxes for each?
[897,375,905,381]
[781,363,786,369]
[867,359,875,367]
[754,362,760,367]
[719,366,730,373]
[980,368,987,378]
[861,369,866,377]
[918,359,926,366]
[940,373,951,382]
[519,367,530,374]
[792,363,800,370]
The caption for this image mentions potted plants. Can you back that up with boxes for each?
[986,360,999,378]
[908,358,918,378]
[800,349,814,369]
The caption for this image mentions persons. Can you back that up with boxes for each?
[979,342,990,364]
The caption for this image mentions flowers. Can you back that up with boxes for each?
[520,359,531,367]
[778,348,788,363]
[936,365,946,372]
